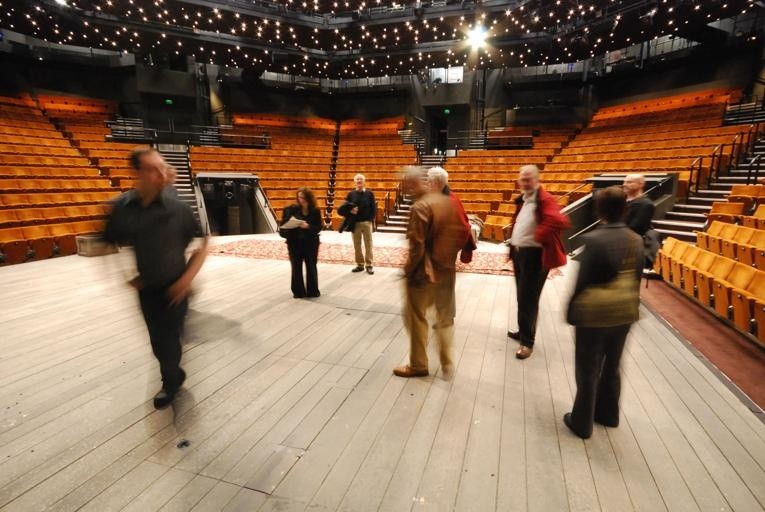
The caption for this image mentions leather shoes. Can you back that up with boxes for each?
[516,345,533,358]
[565,413,589,438]
[352,267,361,272]
[508,329,520,339]
[154,389,174,407]
[367,267,374,274]
[394,365,428,377]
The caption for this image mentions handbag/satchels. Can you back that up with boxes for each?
[574,269,640,328]
[642,229,659,268]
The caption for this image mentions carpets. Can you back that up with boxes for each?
[189,237,566,280]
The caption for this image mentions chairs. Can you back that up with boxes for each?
[185,112,421,230]
[0,91,156,269]
[441,84,765,245]
[653,244,765,347]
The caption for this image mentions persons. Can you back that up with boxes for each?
[277,187,323,299]
[102,143,211,408]
[392,166,456,382]
[562,186,645,440]
[162,161,178,200]
[621,173,656,237]
[503,165,571,359]
[426,167,478,330]
[336,173,377,275]
[641,225,660,268]
